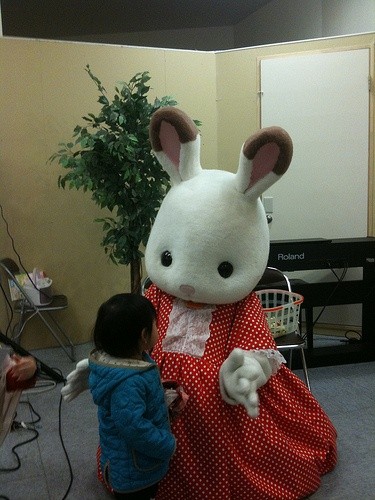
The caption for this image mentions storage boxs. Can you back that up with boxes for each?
[24,267,53,307]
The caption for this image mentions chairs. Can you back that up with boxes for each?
[254,267,311,392]
[0,258,77,363]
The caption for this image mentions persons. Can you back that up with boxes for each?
[0,346,40,447]
[89,293,177,500]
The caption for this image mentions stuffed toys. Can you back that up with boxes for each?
[61,107,338,500]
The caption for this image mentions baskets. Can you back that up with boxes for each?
[22,268,53,306]
[251,288,304,338]
